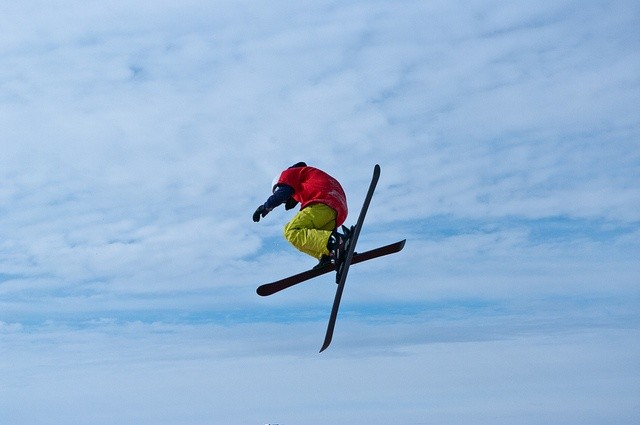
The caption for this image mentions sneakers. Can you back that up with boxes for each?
[314,254,332,270]
[326,232,351,284]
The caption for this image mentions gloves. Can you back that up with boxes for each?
[285,197,298,209]
[253,205,268,222]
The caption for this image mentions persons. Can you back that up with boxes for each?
[252,162,349,274]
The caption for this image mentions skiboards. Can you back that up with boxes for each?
[254,164,408,354]
